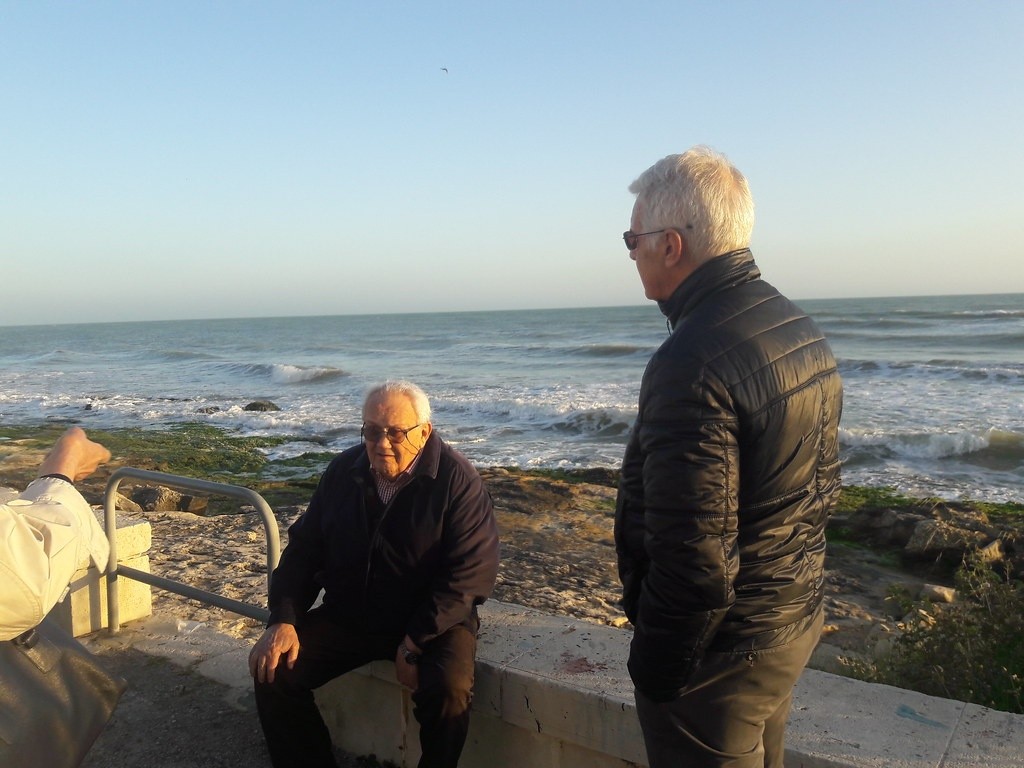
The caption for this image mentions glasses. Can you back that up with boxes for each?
[623,225,693,250]
[361,422,420,443]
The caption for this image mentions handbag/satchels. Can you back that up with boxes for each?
[0,615,129,768]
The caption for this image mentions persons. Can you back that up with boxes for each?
[0,426,111,767]
[614,148,844,768]
[249,382,500,768]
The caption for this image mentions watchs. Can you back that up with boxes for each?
[399,641,422,665]
